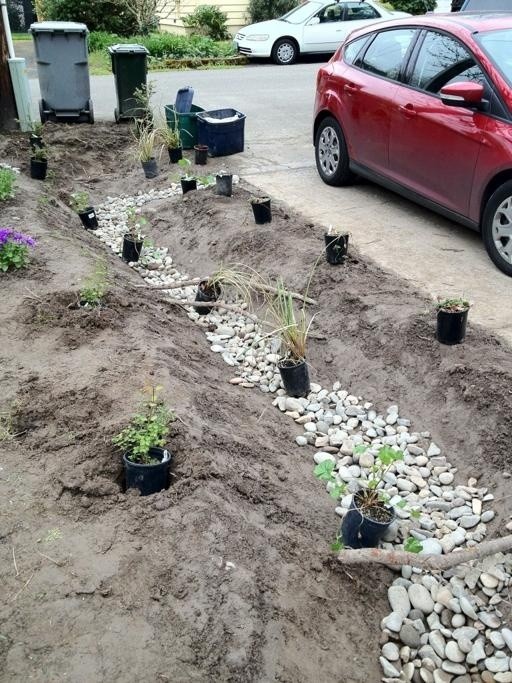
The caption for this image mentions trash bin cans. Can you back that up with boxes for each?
[108,44,153,123]
[28,21,94,124]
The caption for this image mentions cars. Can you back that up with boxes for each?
[313,11,512,274]
[232,0,420,64]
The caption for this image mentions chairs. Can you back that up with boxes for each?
[317,9,337,23]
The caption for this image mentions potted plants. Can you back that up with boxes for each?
[434,294,471,342]
[265,278,312,398]
[111,384,171,495]
[315,440,406,548]
